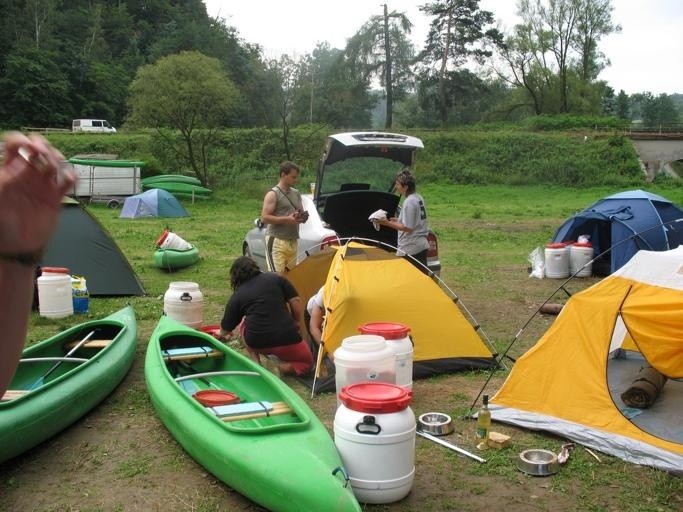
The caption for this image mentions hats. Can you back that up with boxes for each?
[395,167,416,184]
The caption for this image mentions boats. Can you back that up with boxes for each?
[0,306,137,463]
[146,315,361,512]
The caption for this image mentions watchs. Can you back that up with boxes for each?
[1,248,45,264]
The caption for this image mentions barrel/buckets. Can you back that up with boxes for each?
[570,243,594,278]
[164,281,204,330]
[332,334,397,409]
[332,382,417,504]
[544,243,570,279]
[565,240,575,257]
[37,267,74,320]
[356,321,415,396]
[155,230,192,251]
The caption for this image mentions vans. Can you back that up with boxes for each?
[72,119,116,133]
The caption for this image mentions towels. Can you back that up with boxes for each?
[367,208,387,232]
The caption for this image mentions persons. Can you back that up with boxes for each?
[0,130,77,402]
[304,284,328,379]
[261,161,304,274]
[376,168,429,276]
[215,257,314,379]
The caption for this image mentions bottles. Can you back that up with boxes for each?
[476,395,490,447]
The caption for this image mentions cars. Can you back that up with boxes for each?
[240,131,441,282]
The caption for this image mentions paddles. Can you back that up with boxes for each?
[179,360,247,402]
[20,328,101,391]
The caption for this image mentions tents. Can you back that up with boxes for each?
[551,189,683,274]
[120,188,190,218]
[37,195,147,295]
[240,237,509,400]
[463,219,683,475]
